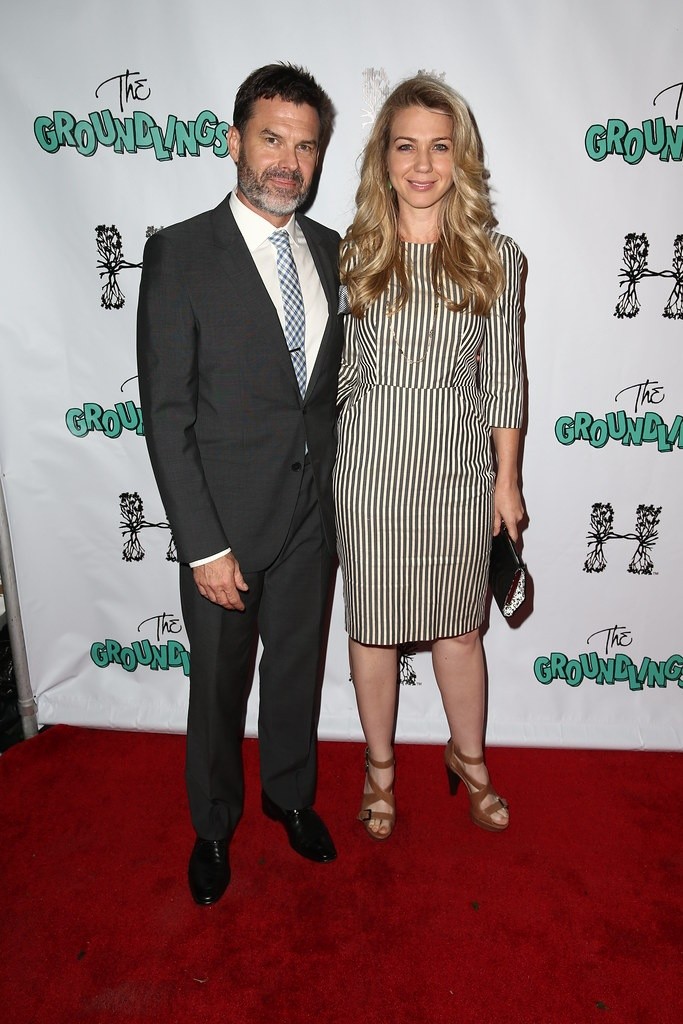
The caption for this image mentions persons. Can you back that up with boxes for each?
[135,62,479,908]
[336,74,532,842]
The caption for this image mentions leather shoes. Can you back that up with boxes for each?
[188,837,231,905]
[261,789,338,864]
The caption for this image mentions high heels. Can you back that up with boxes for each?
[359,745,396,841]
[444,737,510,832]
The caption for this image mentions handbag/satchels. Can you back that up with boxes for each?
[491,524,528,619]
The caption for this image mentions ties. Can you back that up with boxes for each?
[268,229,306,394]
